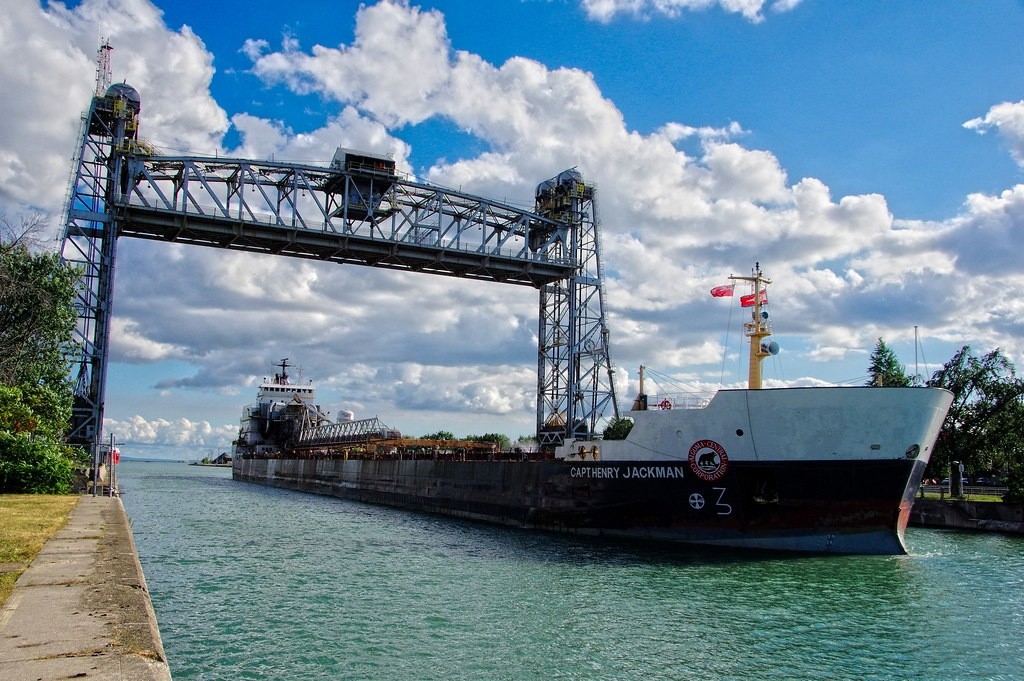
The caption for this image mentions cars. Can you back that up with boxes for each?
[976,477,990,486]
[961,478,969,485]
[942,477,949,485]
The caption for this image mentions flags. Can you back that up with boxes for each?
[742,289,768,306]
[711,284,734,297]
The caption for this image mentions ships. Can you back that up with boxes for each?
[230,356,952,561]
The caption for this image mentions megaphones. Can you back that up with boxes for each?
[763,341,780,356]
